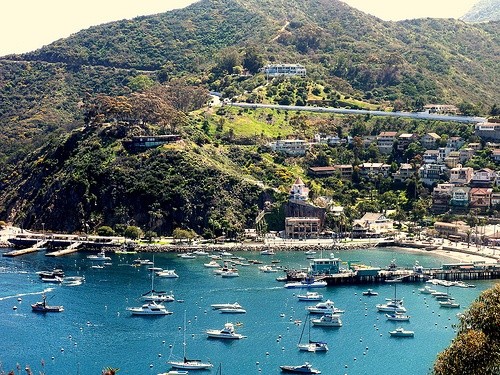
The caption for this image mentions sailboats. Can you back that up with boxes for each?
[165,310,214,370]
[296,311,329,354]
[438,288,460,308]
[375,281,411,322]
[140,254,175,302]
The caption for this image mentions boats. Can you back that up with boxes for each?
[279,361,322,375]
[362,289,379,296]
[297,292,324,301]
[35,249,288,287]
[388,326,414,337]
[31,292,65,312]
[126,300,174,315]
[283,274,328,288]
[205,316,243,340]
[156,370,190,375]
[309,313,343,327]
[211,302,247,313]
[417,278,475,301]
[304,299,344,314]
